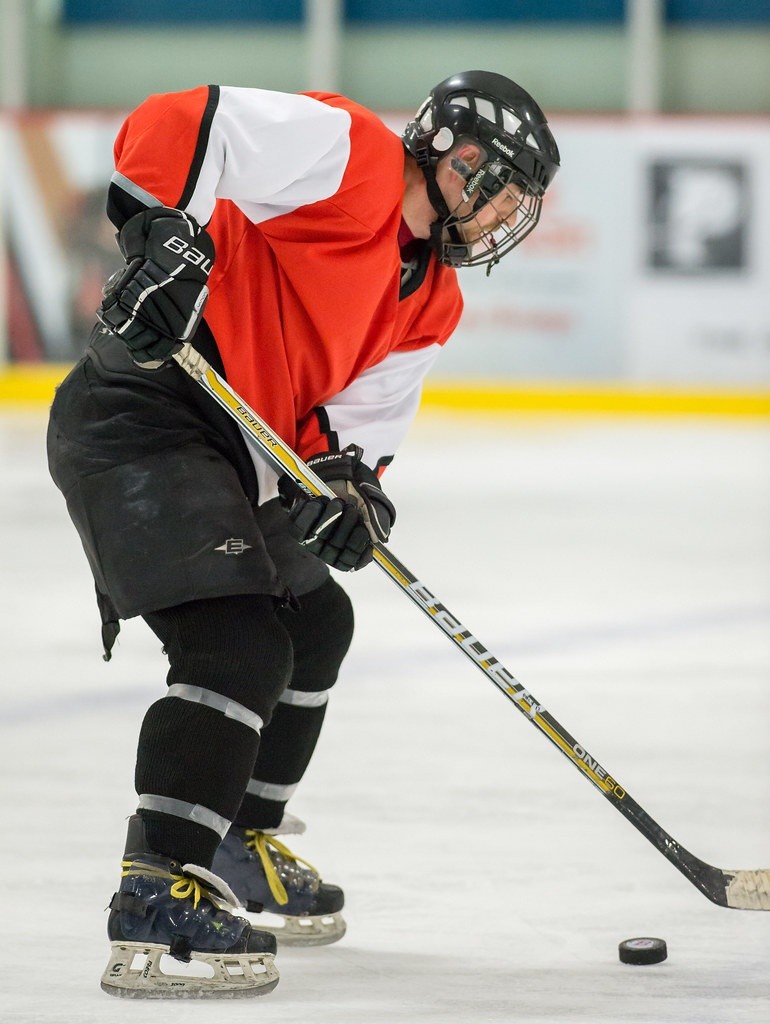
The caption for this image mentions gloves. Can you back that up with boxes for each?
[100,205,216,370]
[276,444,395,571]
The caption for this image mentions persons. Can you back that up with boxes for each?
[44,69,561,1002]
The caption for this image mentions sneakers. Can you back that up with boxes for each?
[100,815,279,999]
[205,815,345,948]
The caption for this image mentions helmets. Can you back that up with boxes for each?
[401,71,560,210]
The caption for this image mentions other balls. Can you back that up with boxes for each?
[616,938,669,968]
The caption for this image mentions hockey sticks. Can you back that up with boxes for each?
[100,266,770,912]
